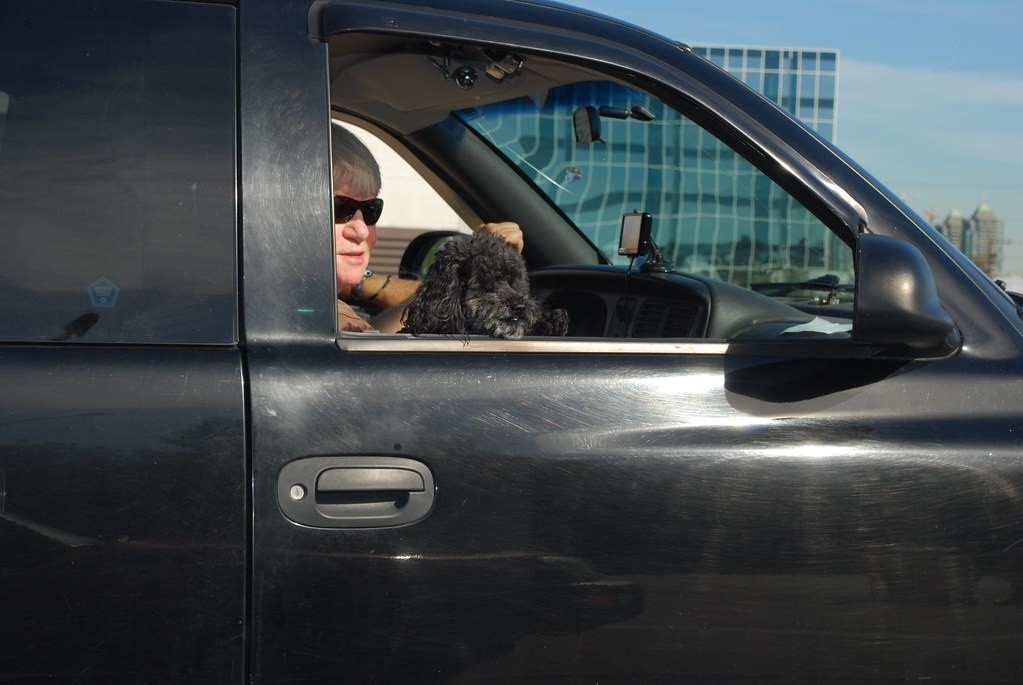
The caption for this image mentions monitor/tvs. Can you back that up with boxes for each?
[618,213,653,256]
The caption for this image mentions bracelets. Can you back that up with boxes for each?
[351,267,391,303]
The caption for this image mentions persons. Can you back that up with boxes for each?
[331,121,524,334]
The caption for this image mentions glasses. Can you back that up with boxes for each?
[333,194,383,226]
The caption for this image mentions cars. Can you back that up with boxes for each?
[0,0,1023,685]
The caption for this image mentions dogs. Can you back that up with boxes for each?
[400,229,570,348]
